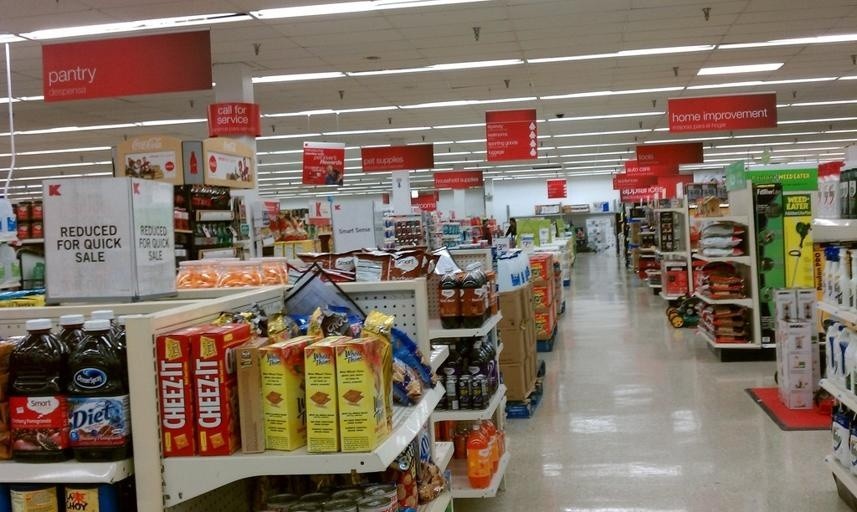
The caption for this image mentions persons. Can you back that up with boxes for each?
[323,165,343,185]
[238,161,244,180]
[127,156,158,179]
[503,218,517,238]
[242,158,250,181]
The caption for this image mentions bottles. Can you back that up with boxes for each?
[437,336,500,410]
[453,421,505,490]
[31,262,44,291]
[436,261,490,329]
[172,183,238,247]
[497,247,532,291]
[7,310,131,461]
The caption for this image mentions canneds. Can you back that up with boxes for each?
[268,483,399,512]
[435,374,489,410]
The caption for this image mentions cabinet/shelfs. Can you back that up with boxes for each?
[617,179,857,512]
[0,184,575,512]
